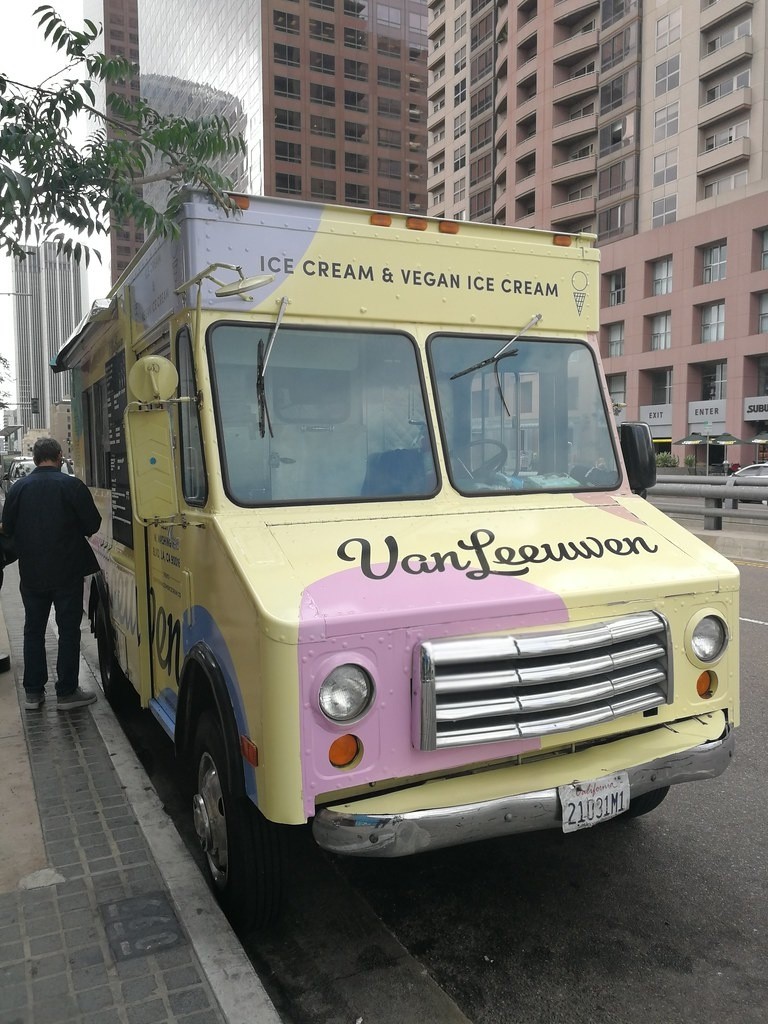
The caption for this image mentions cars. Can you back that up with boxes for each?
[726,463,768,504]
[3,455,76,500]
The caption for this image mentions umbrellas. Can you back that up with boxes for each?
[672,430,768,476]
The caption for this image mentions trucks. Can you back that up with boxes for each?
[47,188,740,935]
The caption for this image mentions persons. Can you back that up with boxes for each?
[722,457,729,466]
[751,461,756,464]
[519,449,538,472]
[0,437,103,711]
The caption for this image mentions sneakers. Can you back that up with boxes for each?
[24,688,47,710]
[56,685,97,711]
